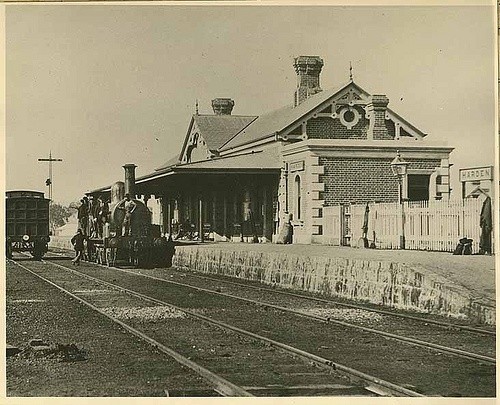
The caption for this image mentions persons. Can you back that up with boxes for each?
[118,193,136,237]
[71,196,110,265]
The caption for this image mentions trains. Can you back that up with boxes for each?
[6,191,50,261]
[76,164,158,269]
